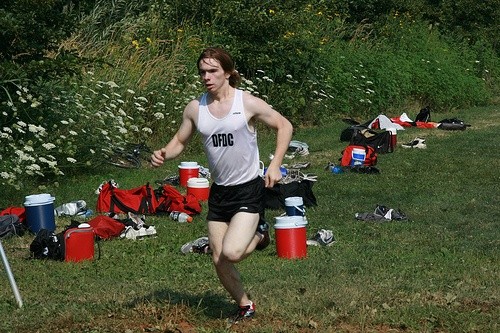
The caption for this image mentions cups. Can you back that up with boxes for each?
[76,209,93,219]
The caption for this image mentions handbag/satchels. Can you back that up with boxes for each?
[153,185,201,215]
[341,106,466,169]
[0,206,26,223]
[0,215,21,240]
[30,228,65,261]
[96,180,157,213]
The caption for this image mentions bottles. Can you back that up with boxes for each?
[169,211,193,223]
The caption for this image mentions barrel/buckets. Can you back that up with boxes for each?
[285,197,306,216]
[178,161,200,187]
[386,128,397,148]
[351,147,366,167]
[24,193,56,235]
[258,161,287,177]
[187,177,209,201]
[274,215,308,260]
[64,223,95,262]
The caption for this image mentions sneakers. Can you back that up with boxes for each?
[254,224,270,250]
[181,236,209,254]
[138,224,157,236]
[119,225,147,239]
[400,136,427,149]
[306,229,336,247]
[233,300,256,323]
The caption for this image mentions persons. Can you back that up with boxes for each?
[149,48,293,324]
